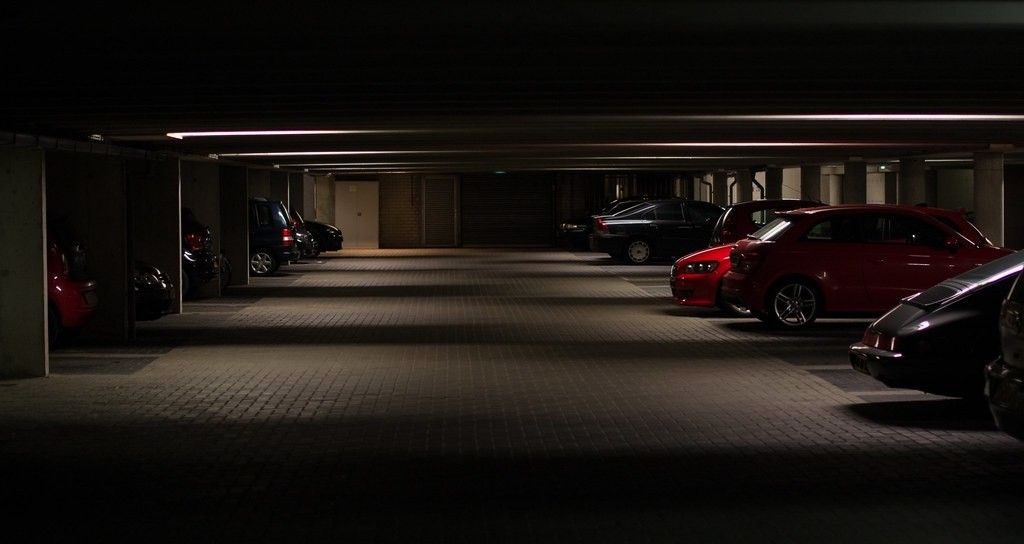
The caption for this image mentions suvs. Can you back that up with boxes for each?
[708,200,826,248]
[670,207,998,318]
[249,197,297,277]
[722,203,1018,333]
[47,182,96,337]
[985,264,1024,440]
[182,207,217,297]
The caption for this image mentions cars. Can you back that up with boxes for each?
[848,249,1024,398]
[588,197,727,265]
[289,208,343,262]
[133,263,176,311]
